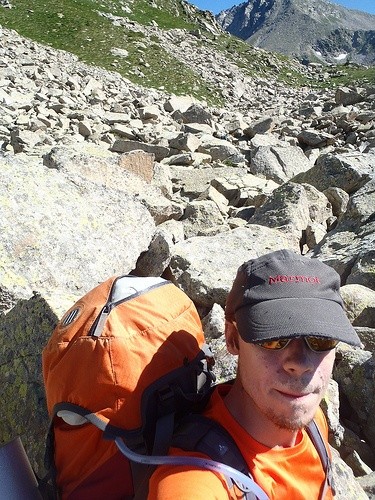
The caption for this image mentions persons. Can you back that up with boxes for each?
[138,250,361,500]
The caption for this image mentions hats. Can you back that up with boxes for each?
[225,249,364,348]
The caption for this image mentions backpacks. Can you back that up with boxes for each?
[41,274,336,499]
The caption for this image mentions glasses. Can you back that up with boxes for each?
[256,335,341,352]
